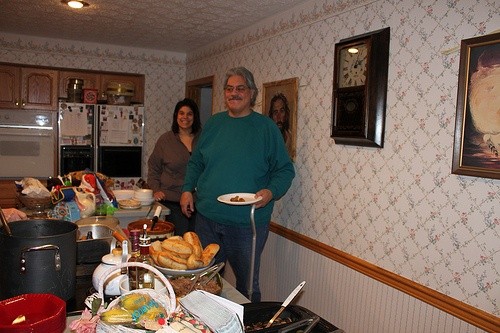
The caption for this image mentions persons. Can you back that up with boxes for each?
[0,208,29,226]
[180,67,297,302]
[147,98,202,235]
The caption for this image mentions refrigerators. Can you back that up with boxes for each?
[56,101,145,184]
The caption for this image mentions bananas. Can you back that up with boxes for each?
[100,293,163,329]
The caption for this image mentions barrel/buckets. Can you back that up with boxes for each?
[0,219,79,304]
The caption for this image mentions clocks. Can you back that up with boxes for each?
[330,26,390,148]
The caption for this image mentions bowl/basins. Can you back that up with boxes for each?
[92,248,147,295]
[112,188,155,205]
[128,218,175,243]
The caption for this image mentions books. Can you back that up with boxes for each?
[178,289,244,333]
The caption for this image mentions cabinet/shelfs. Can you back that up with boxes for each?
[0,64,57,111]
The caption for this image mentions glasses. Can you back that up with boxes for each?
[225,85,250,93]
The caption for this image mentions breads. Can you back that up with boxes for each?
[149,232,220,269]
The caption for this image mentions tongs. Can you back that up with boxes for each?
[183,261,226,289]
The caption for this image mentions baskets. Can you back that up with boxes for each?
[96,262,177,333]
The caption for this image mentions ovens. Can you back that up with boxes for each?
[0,110,56,178]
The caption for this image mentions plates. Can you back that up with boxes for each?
[121,205,142,210]
[150,256,216,278]
[217,192,263,206]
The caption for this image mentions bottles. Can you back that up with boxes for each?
[126,230,155,291]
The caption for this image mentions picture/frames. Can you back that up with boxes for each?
[262,77,299,164]
[451,33,500,181]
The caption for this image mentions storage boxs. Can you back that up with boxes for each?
[82,87,100,104]
[76,223,113,264]
